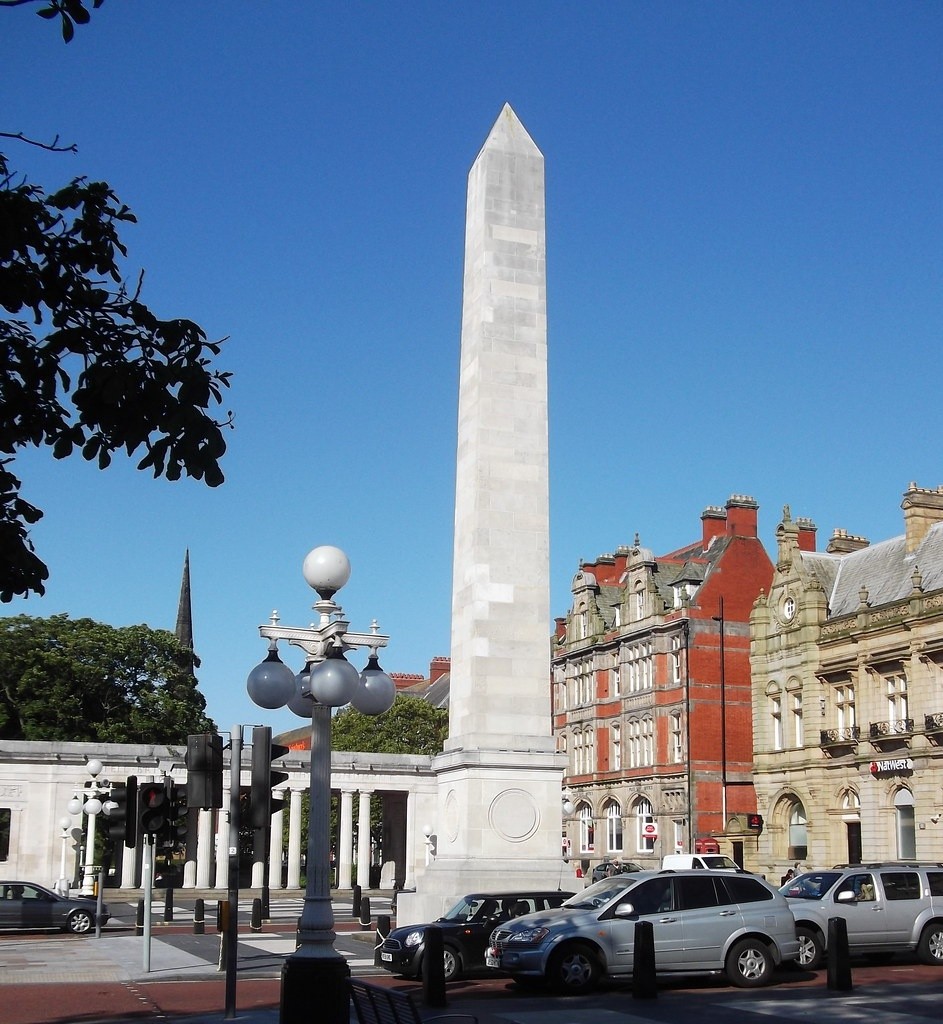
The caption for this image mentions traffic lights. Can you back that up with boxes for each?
[163,776,189,851]
[249,727,290,830]
[138,782,167,836]
[108,774,136,849]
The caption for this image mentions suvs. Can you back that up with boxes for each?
[381,890,580,984]
[773,861,943,973]
[483,868,799,997]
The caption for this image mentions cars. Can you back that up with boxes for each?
[0,879,112,935]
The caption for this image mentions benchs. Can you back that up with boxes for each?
[345,976,477,1024]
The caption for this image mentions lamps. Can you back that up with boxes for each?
[931,813,942,824]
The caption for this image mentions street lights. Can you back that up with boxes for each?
[66,759,118,900]
[54,815,72,900]
[246,545,397,1023]
